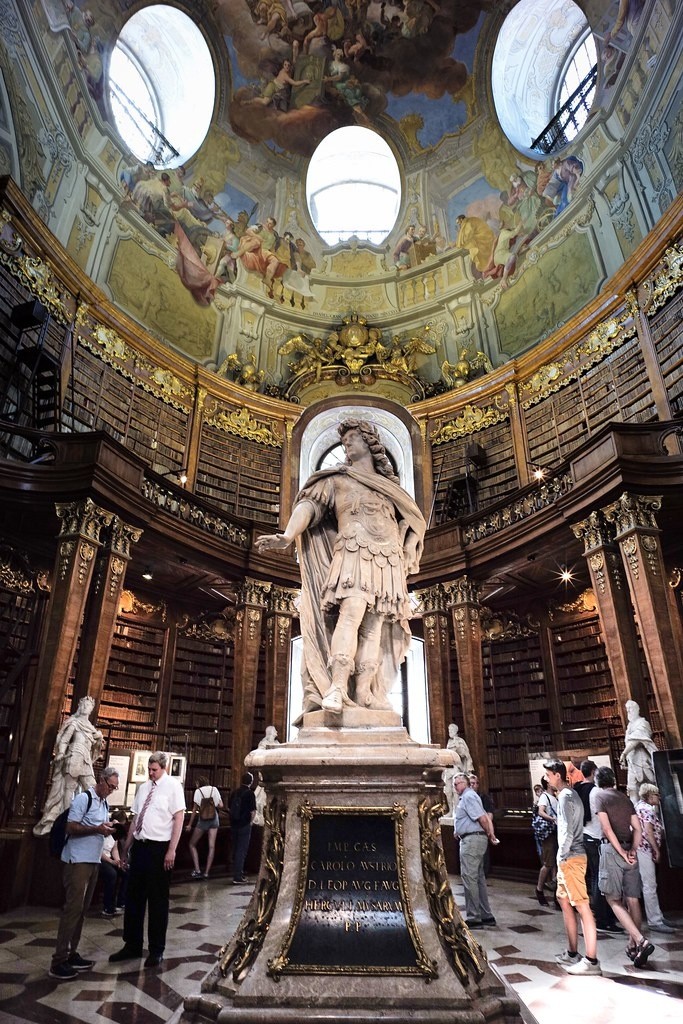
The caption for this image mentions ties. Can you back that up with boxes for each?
[135,781,156,835]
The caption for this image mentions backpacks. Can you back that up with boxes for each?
[48,790,108,860]
[229,789,249,825]
[198,786,215,821]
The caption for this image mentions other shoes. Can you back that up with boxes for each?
[69,958,93,969]
[49,961,79,979]
[185,870,202,878]
[200,874,208,882]
[464,920,483,929]
[534,888,549,906]
[108,946,142,963]
[554,892,562,911]
[482,917,496,926]
[232,876,248,884]
[144,953,163,967]
[101,905,125,916]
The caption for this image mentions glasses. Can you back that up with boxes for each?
[104,778,118,790]
[655,794,660,798]
[454,782,463,787]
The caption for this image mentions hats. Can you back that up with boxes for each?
[543,759,566,781]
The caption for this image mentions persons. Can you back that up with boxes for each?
[49,767,120,979]
[251,726,281,826]
[289,337,335,379]
[441,724,475,816]
[383,335,416,376]
[228,772,257,885]
[185,776,223,880]
[254,418,427,714]
[33,696,103,835]
[532,759,677,975]
[241,771,267,875]
[89,810,130,916]
[453,773,496,929]
[619,700,660,815]
[108,751,187,968]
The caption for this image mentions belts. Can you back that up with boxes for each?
[602,838,630,845]
[458,831,487,841]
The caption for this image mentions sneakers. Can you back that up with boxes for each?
[554,950,602,975]
[596,918,676,967]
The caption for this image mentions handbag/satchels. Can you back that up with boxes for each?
[652,850,657,862]
[532,793,558,842]
